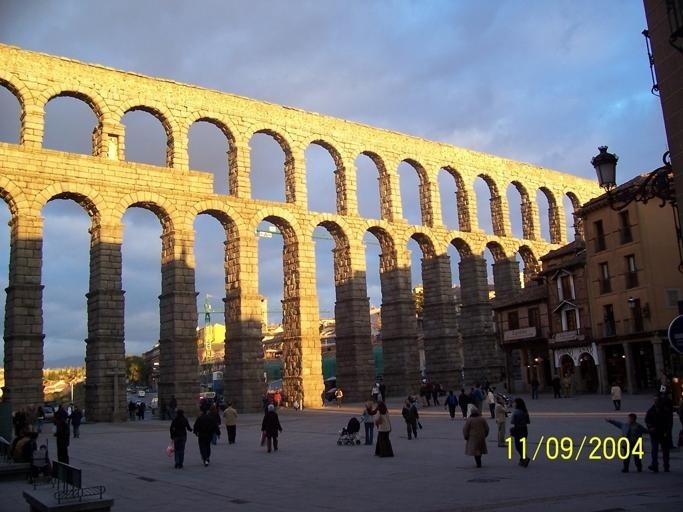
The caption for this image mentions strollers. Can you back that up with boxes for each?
[336,412,365,445]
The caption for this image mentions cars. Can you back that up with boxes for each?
[126,368,426,407]
[43,401,85,421]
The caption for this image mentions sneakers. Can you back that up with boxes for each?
[621,464,670,473]
[365,431,530,467]
[174,447,278,469]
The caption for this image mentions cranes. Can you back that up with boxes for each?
[197,294,332,373]
[257,224,381,246]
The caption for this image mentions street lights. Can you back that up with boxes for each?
[591,143,683,282]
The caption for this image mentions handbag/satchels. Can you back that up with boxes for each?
[261,432,267,446]
[418,422,422,429]
[659,384,667,393]
[166,446,175,457]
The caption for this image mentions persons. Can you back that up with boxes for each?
[159,389,304,470]
[128,400,136,421]
[561,373,575,399]
[604,378,683,474]
[530,374,541,399]
[6,395,85,466]
[138,401,146,422]
[549,373,560,397]
[152,401,156,415]
[362,381,530,470]
[335,387,344,407]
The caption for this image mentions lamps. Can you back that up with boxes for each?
[591,144,676,212]
